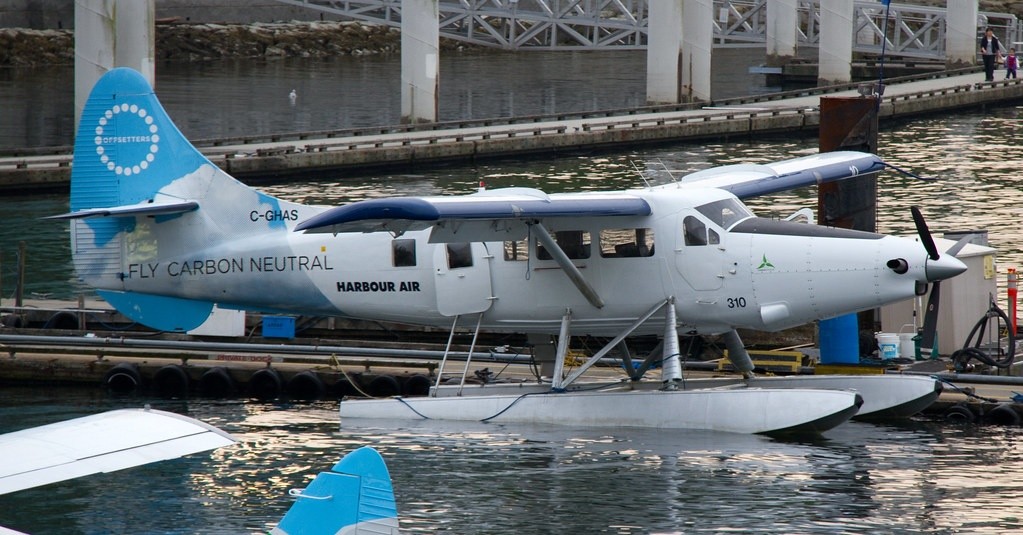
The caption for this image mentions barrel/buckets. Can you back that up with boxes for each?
[818,311,860,365]
[875,333,899,360]
[896,332,919,359]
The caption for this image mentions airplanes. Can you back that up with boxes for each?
[32,68,968,441]
[0,405,404,535]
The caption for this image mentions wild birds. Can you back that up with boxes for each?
[288,88,296,100]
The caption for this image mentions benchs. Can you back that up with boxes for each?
[615,241,641,257]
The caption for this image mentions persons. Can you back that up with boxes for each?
[980,27,1000,81]
[999,48,1020,86]
